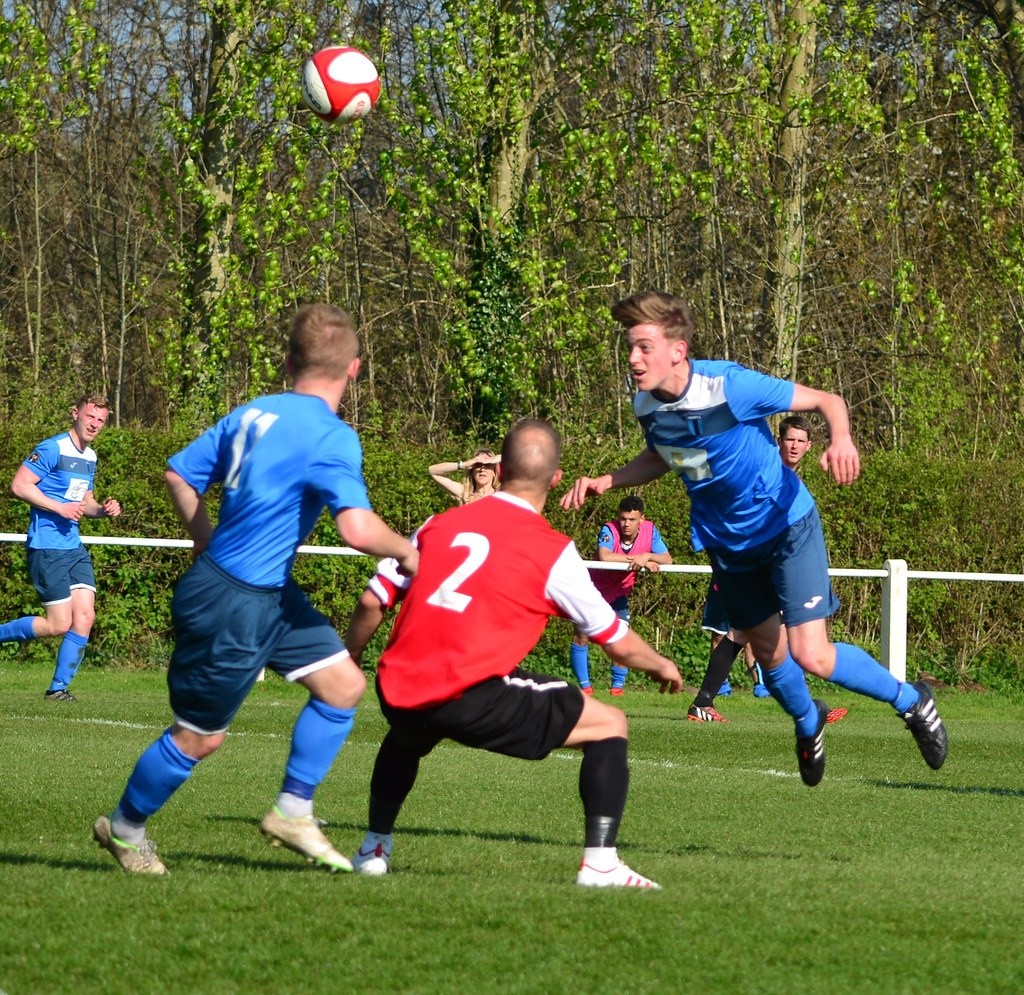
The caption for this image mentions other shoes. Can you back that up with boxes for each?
[583,686,595,696]
[609,687,624,697]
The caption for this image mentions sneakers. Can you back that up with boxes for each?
[687,702,729,723]
[828,708,848,723]
[794,699,829,786]
[353,843,389,875]
[575,859,663,890]
[43,689,78,702]
[93,813,173,877]
[894,680,947,770]
[259,803,355,873]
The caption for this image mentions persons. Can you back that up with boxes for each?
[687,417,847,723]
[571,495,672,699]
[345,418,683,891]
[0,394,121,703]
[559,293,949,787]
[429,450,502,507]
[94,303,420,877]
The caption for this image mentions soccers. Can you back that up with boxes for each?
[299,43,382,124]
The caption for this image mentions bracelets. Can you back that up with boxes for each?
[458,461,463,472]
